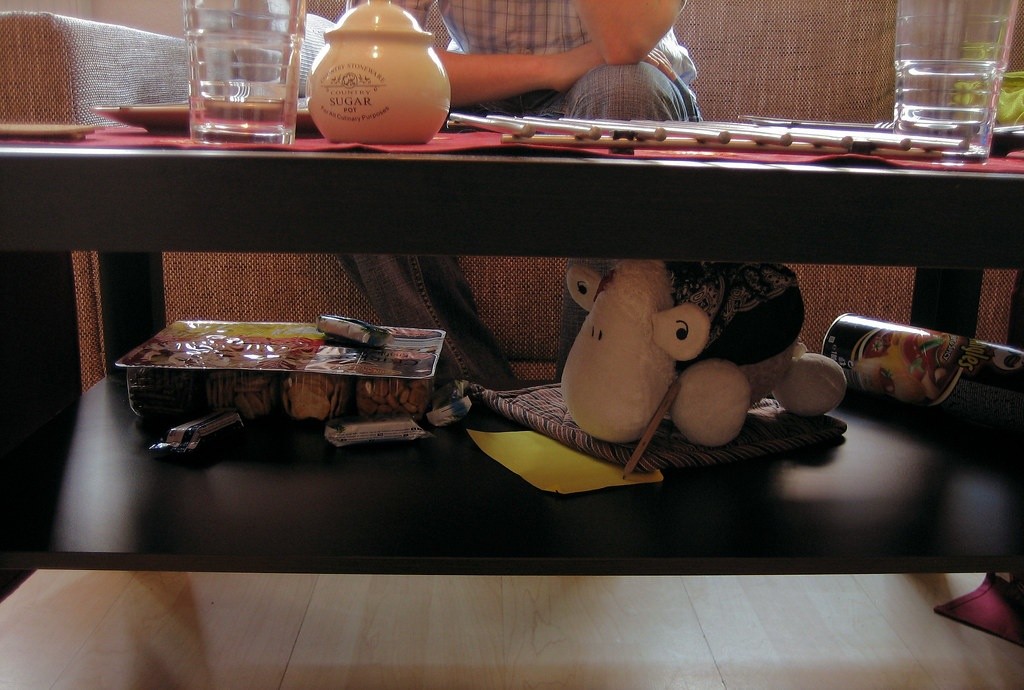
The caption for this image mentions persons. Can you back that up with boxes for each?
[334,0,703,427]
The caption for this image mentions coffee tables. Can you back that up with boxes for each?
[0,126,1024,602]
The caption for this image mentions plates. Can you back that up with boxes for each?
[0,123,96,141]
[90,103,318,136]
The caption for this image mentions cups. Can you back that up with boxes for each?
[893,0,1019,164]
[181,0,307,145]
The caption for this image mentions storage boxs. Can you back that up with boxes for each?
[114,317,447,422]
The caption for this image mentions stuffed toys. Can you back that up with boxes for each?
[562,259,848,447]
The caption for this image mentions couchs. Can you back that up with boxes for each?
[0,0,1024,392]
